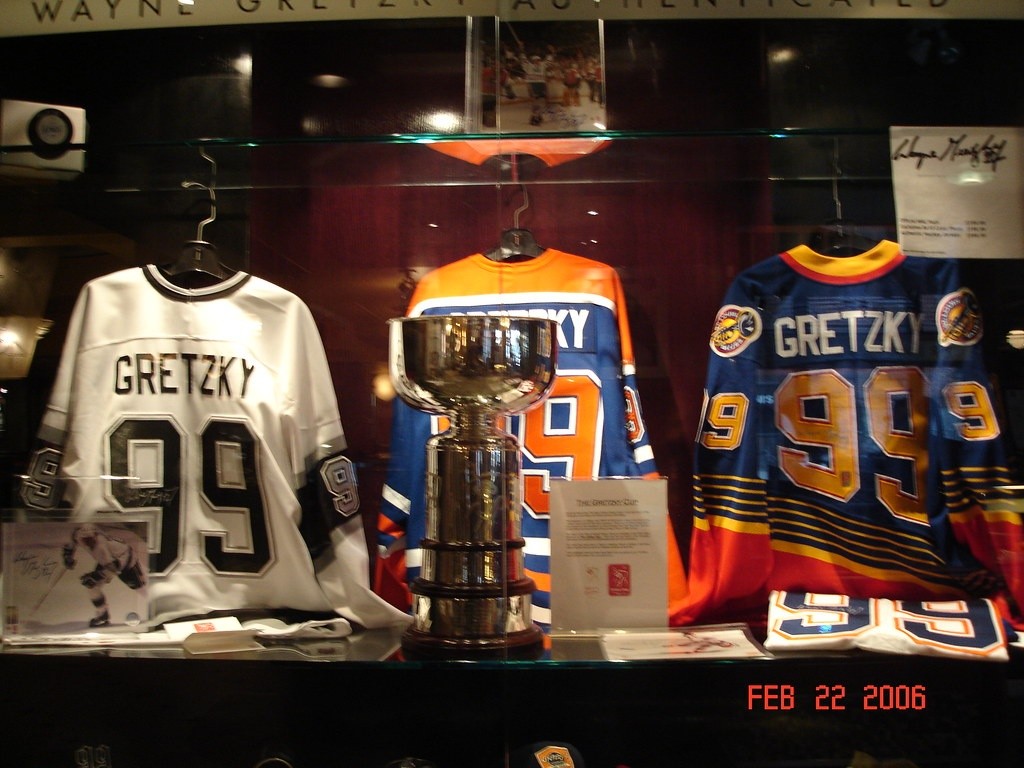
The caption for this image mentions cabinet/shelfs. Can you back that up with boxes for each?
[0,123,1024,768]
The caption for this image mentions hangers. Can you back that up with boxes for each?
[484,180,548,262]
[159,180,236,278]
[809,170,878,255]
